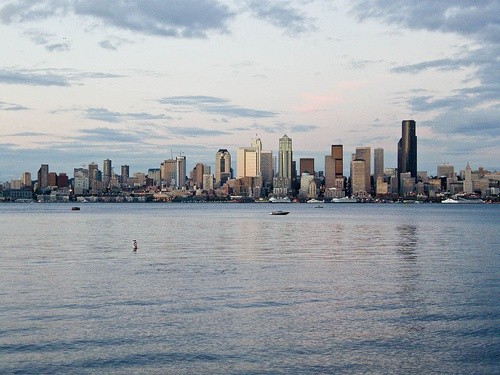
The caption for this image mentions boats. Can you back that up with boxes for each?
[307,199,325,204]
[270,210,289,215]
[229,194,256,203]
[268,196,292,203]
[331,192,499,204]
